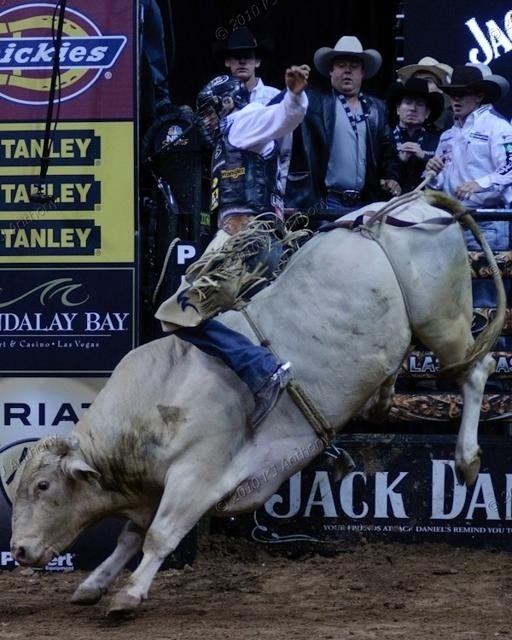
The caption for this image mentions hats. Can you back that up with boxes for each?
[438,67,501,104]
[220,27,261,60]
[386,77,444,124]
[313,36,383,80]
[464,62,510,98]
[398,56,455,87]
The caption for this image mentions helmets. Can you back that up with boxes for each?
[198,74,250,109]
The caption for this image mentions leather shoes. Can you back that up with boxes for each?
[247,364,294,431]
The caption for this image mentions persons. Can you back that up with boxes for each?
[141,35,512,256]
[159,63,310,431]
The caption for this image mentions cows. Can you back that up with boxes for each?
[6,179,508,622]
